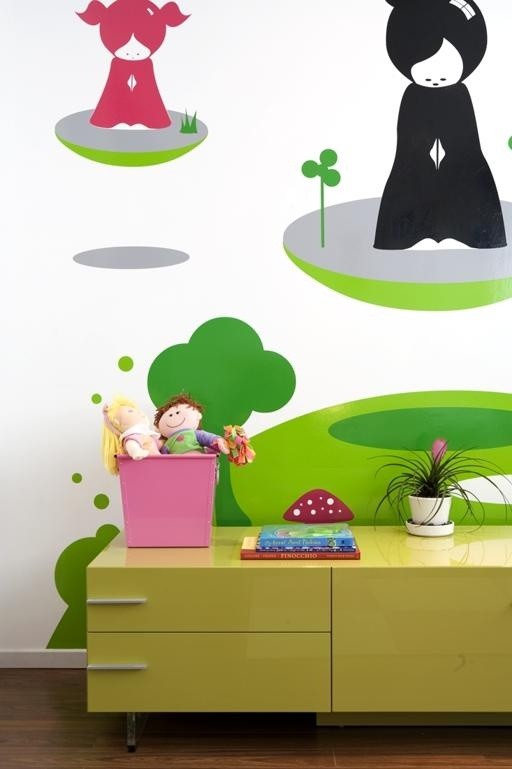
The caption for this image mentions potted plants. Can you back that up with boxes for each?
[367,444,511,539]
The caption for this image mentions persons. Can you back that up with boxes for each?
[102,396,162,476]
[153,392,231,455]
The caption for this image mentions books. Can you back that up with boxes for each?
[241,523,360,560]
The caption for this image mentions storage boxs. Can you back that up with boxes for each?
[114,450,219,549]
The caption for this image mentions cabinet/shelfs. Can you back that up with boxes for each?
[86,524,512,756]
